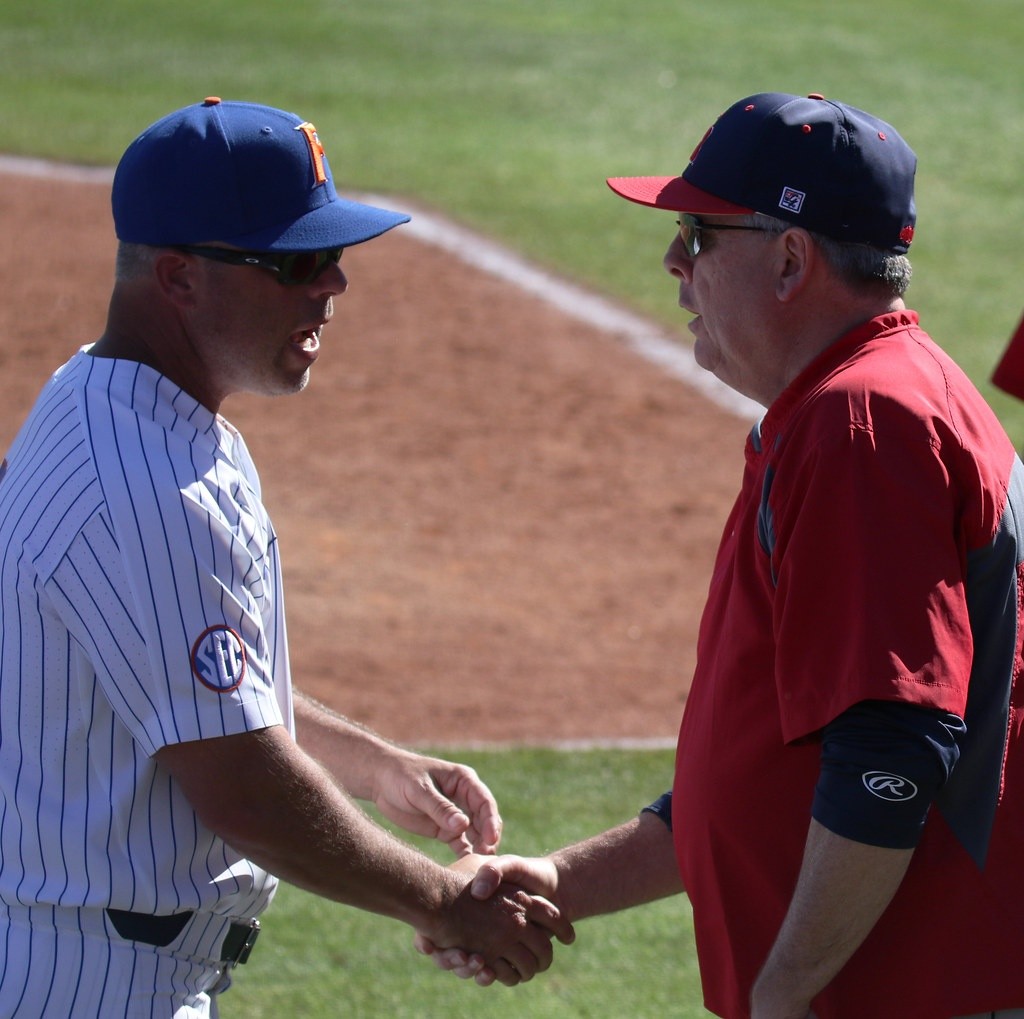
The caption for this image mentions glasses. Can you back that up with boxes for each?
[675,213,786,258]
[180,243,344,289]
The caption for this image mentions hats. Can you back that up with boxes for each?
[605,91,920,255]
[111,96,413,252]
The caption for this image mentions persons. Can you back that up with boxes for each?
[0,96,575,1019]
[419,93,1024,1019]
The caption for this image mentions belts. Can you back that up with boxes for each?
[106,907,262,969]
